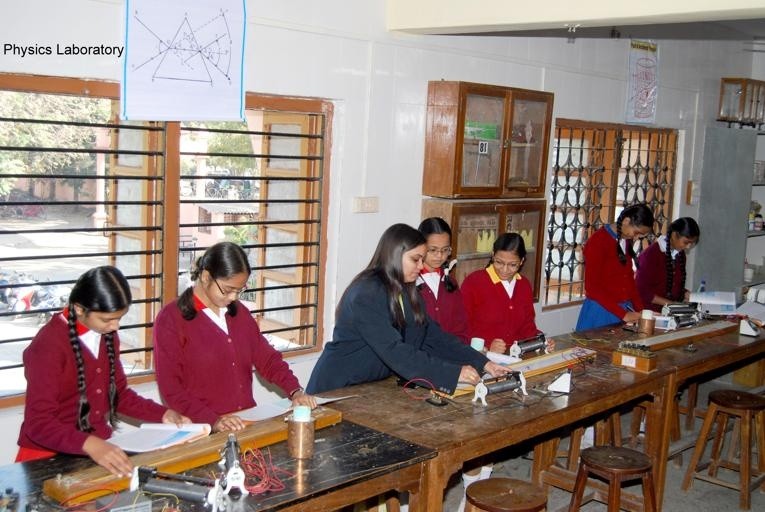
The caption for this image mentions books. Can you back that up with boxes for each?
[105,424,212,454]
[689,291,737,315]
[228,395,360,422]
[653,316,676,329]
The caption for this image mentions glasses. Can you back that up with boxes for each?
[492,256,521,269]
[426,246,452,255]
[211,274,248,296]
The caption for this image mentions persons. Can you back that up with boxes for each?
[460,232,555,354]
[14,266,193,478]
[305,223,512,395]
[417,217,466,344]
[636,217,700,313]
[153,242,317,432]
[575,204,653,331]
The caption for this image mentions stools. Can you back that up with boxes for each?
[569,445,657,512]
[682,389,765,511]
[464,478,549,512]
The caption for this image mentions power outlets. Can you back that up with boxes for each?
[354,197,380,213]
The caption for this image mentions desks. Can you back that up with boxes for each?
[307,338,677,512]
[1,398,439,512]
[552,317,765,511]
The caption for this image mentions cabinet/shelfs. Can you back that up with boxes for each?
[718,77,765,121]
[421,198,547,303]
[743,184,765,287]
[422,80,555,199]
